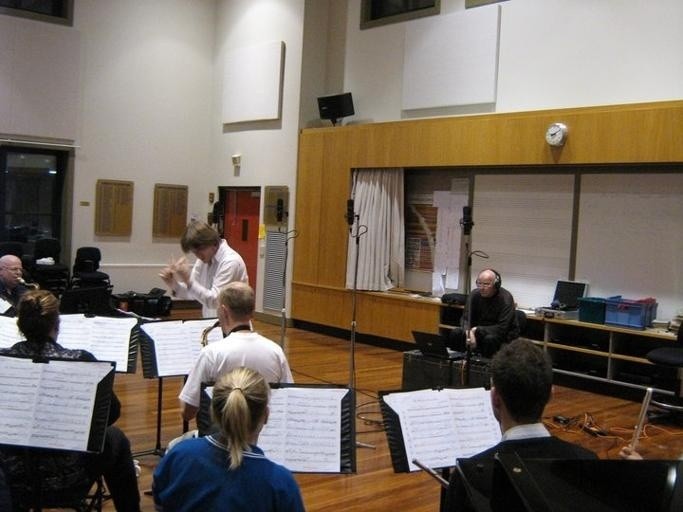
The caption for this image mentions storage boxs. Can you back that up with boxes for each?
[533,278,588,320]
[576,296,607,325]
[604,294,656,329]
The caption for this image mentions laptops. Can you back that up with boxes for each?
[411,331,467,360]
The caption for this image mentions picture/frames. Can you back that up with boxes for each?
[464,0,509,9]
[152,183,187,241]
[358,0,440,28]
[95,179,134,236]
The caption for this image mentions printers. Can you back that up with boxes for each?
[111,287,172,317]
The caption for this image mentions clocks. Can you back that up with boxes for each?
[544,122,567,147]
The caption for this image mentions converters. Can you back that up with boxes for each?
[580,422,601,435]
[554,415,567,423]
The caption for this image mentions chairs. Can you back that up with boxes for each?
[513,308,526,331]
[19,236,112,317]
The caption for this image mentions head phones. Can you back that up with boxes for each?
[476,268,501,290]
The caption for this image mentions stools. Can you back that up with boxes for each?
[20,473,106,512]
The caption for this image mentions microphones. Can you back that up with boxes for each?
[463,206,471,243]
[277,199,283,228]
[347,199,354,233]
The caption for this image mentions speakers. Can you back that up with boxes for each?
[402,348,491,390]
[316,92,354,121]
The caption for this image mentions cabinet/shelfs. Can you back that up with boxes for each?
[438,302,682,408]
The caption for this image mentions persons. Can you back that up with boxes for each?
[445,340,600,511]
[0,290,140,512]
[158,221,249,320]
[166,282,295,455]
[449,270,521,358]
[152,367,306,512]
[0,254,29,317]
